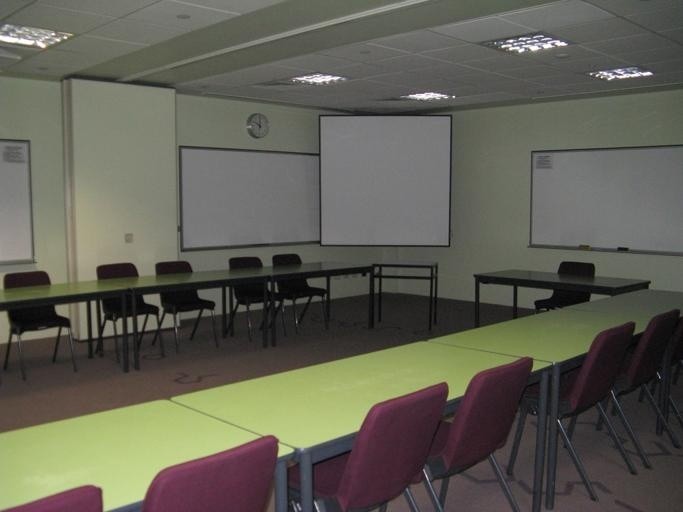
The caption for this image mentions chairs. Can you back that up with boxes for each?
[534,260,596,314]
[507,321,639,502]
[141,435,280,511]
[1,270,79,380]
[94,262,165,366]
[597,308,682,451]
[223,256,287,340]
[152,260,219,350]
[271,253,329,335]
[287,382,449,512]
[414,355,535,511]
[0,484,104,511]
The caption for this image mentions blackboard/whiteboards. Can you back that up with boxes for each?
[530,145,683,256]
[317,114,451,248]
[180,145,319,252]
[0,138,36,266]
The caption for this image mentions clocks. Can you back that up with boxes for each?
[244,111,270,140]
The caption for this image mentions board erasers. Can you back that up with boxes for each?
[579,245,591,251]
[616,247,631,252]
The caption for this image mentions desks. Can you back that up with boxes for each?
[169,341,553,511]
[0,262,374,375]
[473,268,651,327]
[372,259,439,331]
[558,289,681,436]
[428,307,654,511]
[1,398,296,512]
